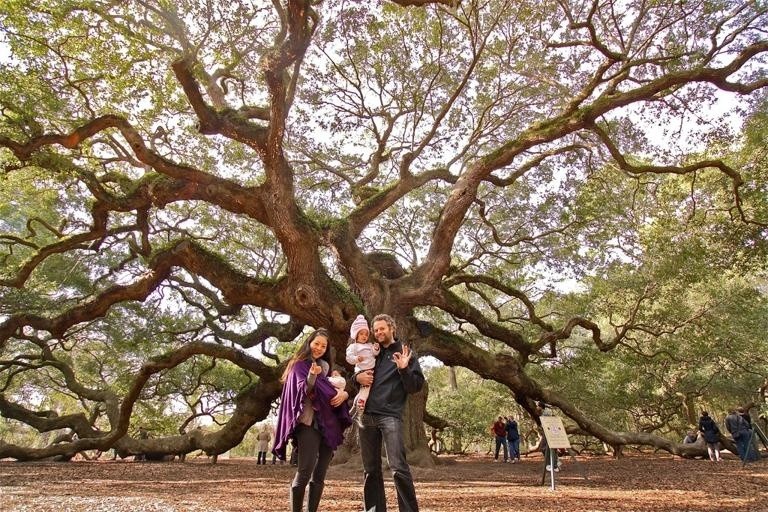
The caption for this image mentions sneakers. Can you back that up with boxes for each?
[347,408,366,430]
[546,465,562,473]
[494,459,520,464]
[257,462,284,465]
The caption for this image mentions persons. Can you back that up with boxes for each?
[488,400,564,473]
[255,424,271,465]
[345,313,381,430]
[272,455,287,464]
[351,314,424,512]
[680,408,758,464]
[272,327,353,512]
[326,368,346,391]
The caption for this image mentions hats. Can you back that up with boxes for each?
[350,315,370,339]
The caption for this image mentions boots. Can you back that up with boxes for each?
[289,483,305,512]
[307,482,324,512]
